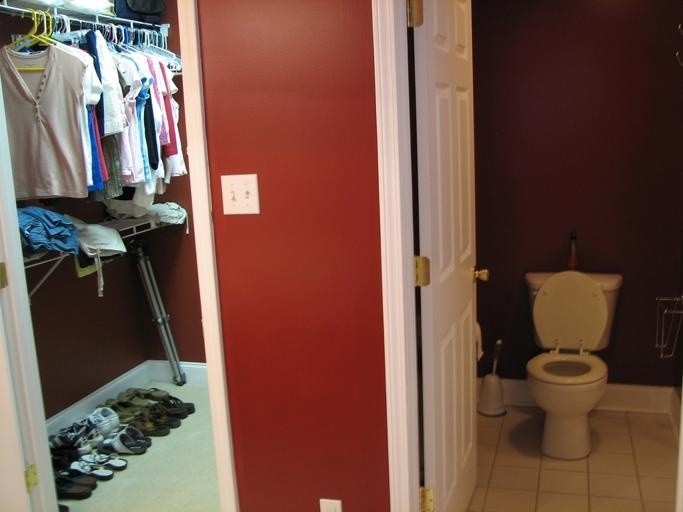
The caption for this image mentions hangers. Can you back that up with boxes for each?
[0,5,182,78]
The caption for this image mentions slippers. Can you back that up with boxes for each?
[48,387,194,499]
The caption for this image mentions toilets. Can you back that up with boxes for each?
[526,271,625,460]
